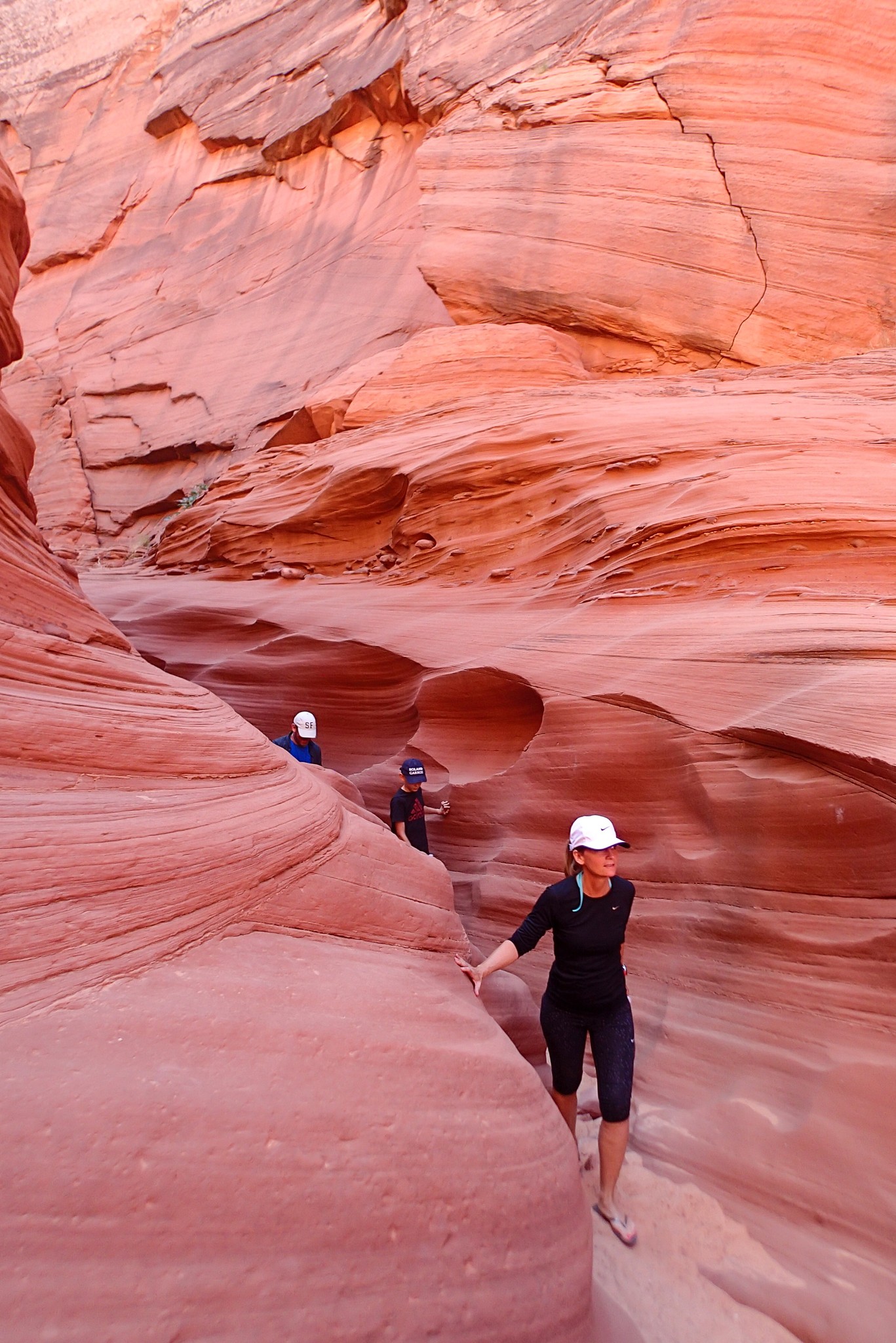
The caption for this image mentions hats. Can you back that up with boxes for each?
[294,711,316,738]
[400,758,427,783]
[568,814,631,851]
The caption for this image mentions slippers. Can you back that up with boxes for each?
[593,1203,637,1246]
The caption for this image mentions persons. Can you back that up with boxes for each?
[389,757,451,856]
[454,813,638,1246]
[272,711,323,766]
